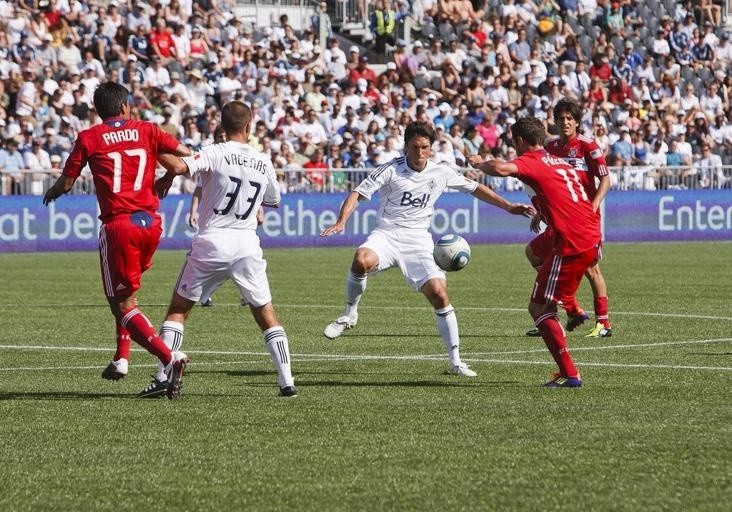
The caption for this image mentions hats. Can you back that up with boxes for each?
[0,0,732,194]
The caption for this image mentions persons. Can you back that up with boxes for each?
[41,82,191,401]
[190,176,264,309]
[136,101,298,397]
[1,0,732,199]
[463,119,604,389]
[318,121,538,377]
[522,98,612,338]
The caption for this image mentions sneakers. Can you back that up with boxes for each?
[136,374,168,399]
[277,386,298,398]
[165,350,190,401]
[447,362,479,378]
[565,308,589,332]
[101,357,129,382]
[324,312,358,339]
[584,322,613,338]
[542,370,583,387]
[525,327,544,337]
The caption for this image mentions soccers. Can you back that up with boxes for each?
[435,234,470,271]
